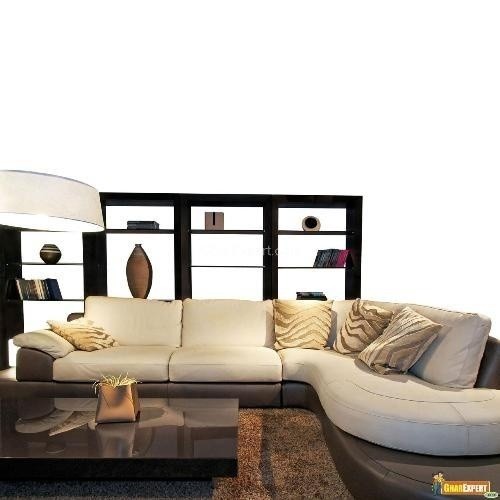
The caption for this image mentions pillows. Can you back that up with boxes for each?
[14,408,95,436]
[328,297,395,362]
[271,298,336,352]
[45,316,120,353]
[357,304,446,377]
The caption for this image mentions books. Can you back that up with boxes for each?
[15,278,64,301]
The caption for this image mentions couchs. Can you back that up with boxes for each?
[15,335,500,499]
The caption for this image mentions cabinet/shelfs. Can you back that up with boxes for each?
[0,192,365,337]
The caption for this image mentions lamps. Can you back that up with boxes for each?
[0,166,104,240]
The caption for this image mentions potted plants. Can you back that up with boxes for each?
[93,370,140,425]
